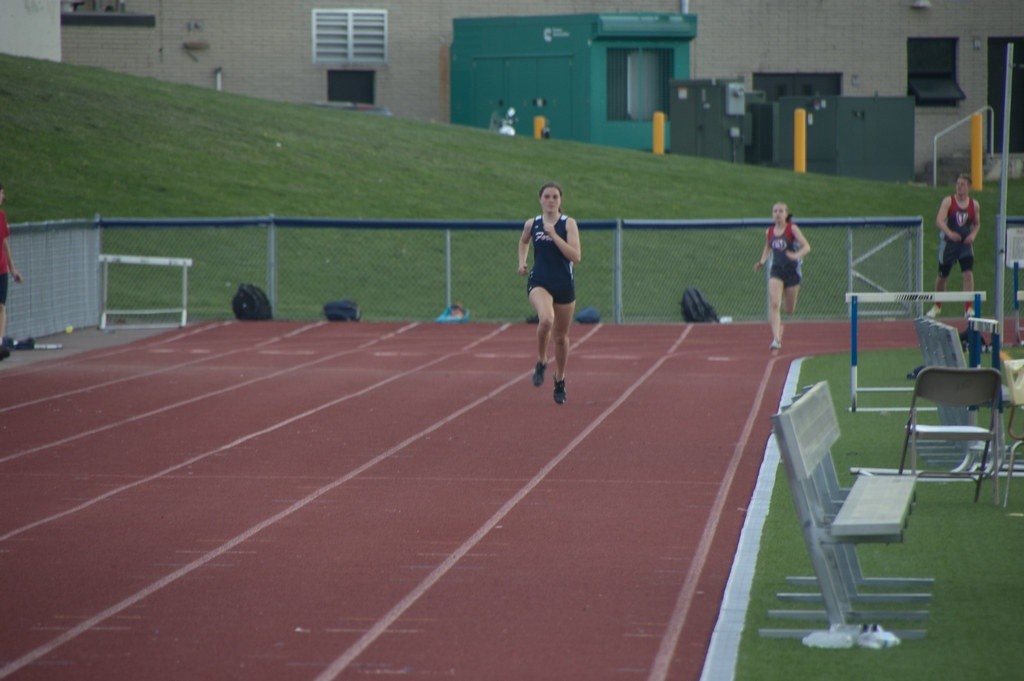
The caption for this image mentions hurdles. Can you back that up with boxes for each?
[844,289,1007,478]
[97,253,194,331]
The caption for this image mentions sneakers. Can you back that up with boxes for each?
[553,371,566,404]
[533,362,547,386]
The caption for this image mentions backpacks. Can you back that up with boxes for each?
[233,281,272,322]
[324,300,362,321]
[680,288,719,323]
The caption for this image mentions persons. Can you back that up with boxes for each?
[754,203,810,349]
[927,175,980,317]
[518,183,580,404]
[0,181,22,361]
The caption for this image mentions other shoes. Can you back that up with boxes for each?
[927,305,941,318]
[964,307,975,318]
[0,346,10,361]
[770,340,781,349]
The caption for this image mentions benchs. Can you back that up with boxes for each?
[758,380,935,640]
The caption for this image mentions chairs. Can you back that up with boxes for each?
[898,365,1024,507]
